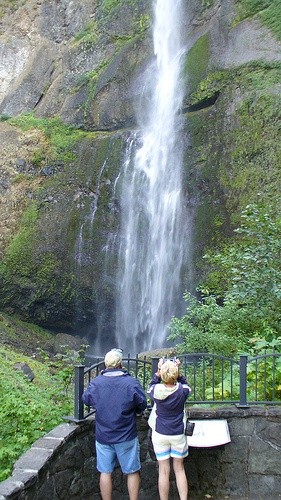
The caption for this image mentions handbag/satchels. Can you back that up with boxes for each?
[186,422,195,435]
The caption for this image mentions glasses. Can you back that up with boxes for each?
[162,358,174,362]
[111,347,123,353]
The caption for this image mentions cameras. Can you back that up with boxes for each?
[164,359,175,363]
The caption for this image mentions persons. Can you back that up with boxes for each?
[148,358,191,498]
[81,348,147,500]
